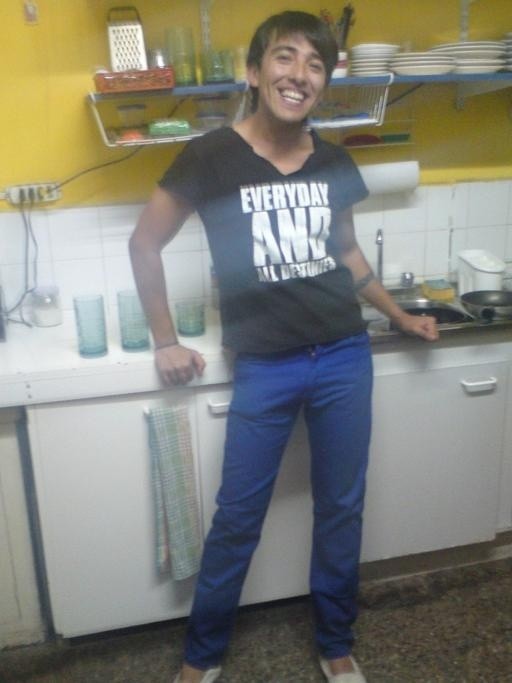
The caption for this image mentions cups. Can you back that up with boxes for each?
[73,293,107,357]
[165,25,198,86]
[118,290,150,350]
[177,302,206,335]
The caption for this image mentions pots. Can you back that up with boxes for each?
[459,289,512,320]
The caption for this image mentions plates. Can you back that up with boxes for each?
[429,41,508,74]
[505,32,512,73]
[352,43,401,75]
[386,50,457,75]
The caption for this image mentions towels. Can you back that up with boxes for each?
[144,406,209,584]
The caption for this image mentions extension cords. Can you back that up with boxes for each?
[9,181,59,205]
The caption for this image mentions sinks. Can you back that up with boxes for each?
[381,300,474,335]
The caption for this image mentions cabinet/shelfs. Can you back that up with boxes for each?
[199,381,316,609]
[2,405,50,652]
[357,319,512,568]
[21,389,200,639]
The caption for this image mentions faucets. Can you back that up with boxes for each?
[373,229,384,293]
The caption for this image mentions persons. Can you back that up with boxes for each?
[124,9,440,682]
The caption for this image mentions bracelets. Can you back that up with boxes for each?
[151,340,180,352]
[349,264,377,298]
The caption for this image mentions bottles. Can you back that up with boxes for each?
[33,286,62,326]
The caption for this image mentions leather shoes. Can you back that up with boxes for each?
[173,665,222,682]
[319,651,364,683]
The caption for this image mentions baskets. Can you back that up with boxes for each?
[95,63,174,92]
